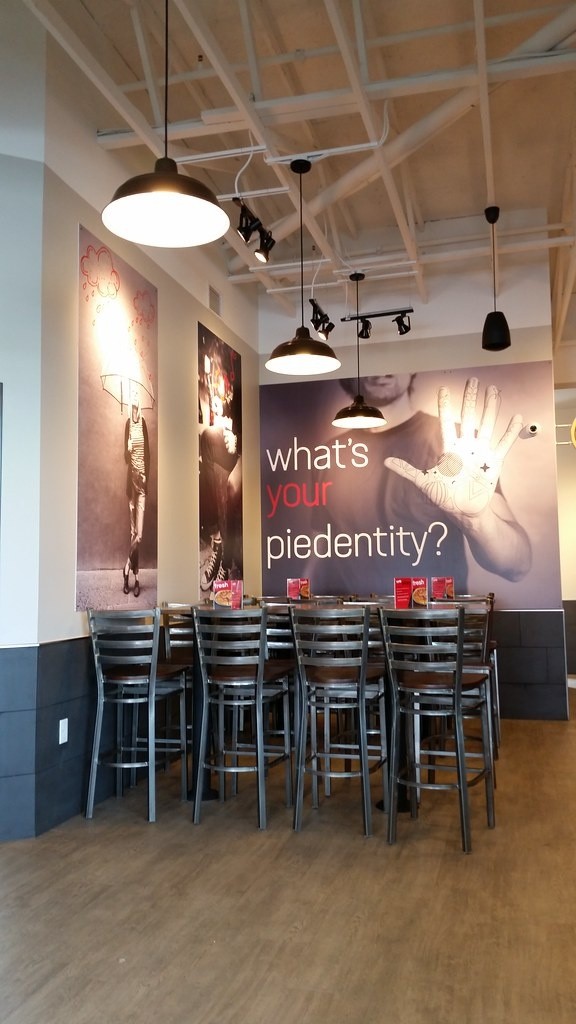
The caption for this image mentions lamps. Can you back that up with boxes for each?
[390,310,415,334]
[482,206,511,351]
[305,299,335,341]
[331,271,386,429]
[358,319,372,339]
[236,199,262,242]
[254,229,276,264]
[264,160,342,375]
[100,5,232,248]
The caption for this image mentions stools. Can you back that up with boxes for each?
[84,592,502,856]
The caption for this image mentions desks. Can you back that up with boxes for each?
[152,600,492,814]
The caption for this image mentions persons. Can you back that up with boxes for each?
[200,355,244,592]
[122,395,152,596]
[301,374,532,599]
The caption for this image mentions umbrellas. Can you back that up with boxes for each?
[100,352,155,452]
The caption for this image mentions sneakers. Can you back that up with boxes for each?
[201,544,223,591]
[211,561,231,594]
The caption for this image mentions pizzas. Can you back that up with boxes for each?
[446,586,454,597]
[214,590,232,606]
[412,588,427,605]
[300,586,309,598]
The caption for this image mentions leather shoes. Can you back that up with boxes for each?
[133,580,140,597]
[122,566,130,594]
